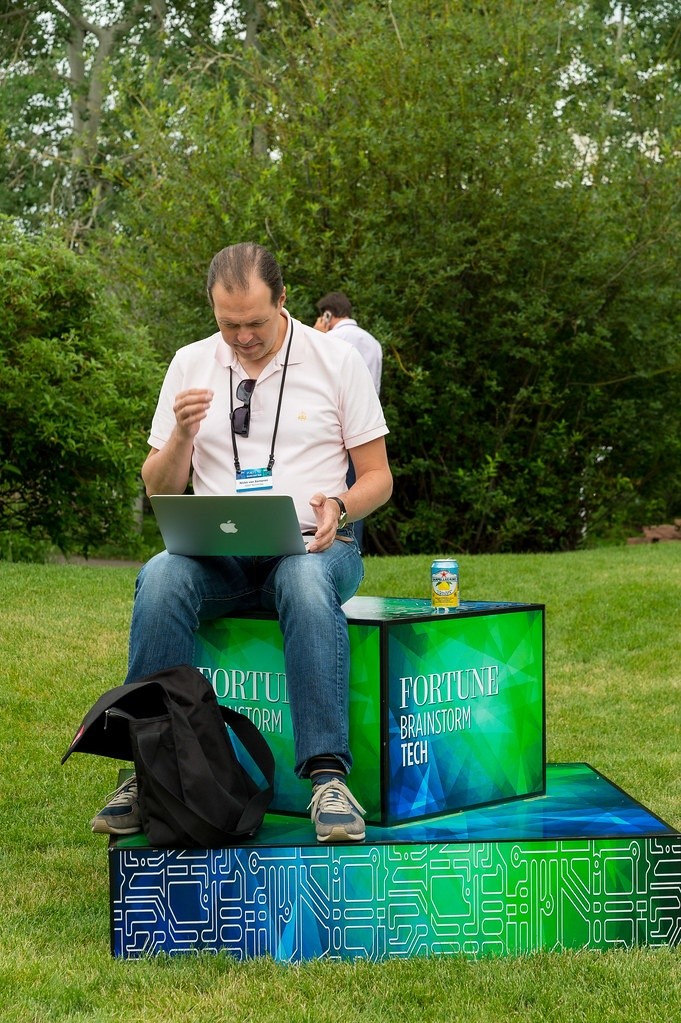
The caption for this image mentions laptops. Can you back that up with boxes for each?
[149,494,316,556]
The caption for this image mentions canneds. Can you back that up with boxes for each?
[431,557,459,610]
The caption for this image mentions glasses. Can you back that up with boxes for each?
[231,378,256,438]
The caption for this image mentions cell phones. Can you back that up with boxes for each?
[322,310,332,325]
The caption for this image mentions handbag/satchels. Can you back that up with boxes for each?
[61,663,275,849]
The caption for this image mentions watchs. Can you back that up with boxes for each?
[328,497,348,530]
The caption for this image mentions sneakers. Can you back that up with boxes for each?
[306,777,366,842]
[90,772,140,834]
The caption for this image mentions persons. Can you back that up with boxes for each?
[312,292,382,554]
[88,242,393,841]
